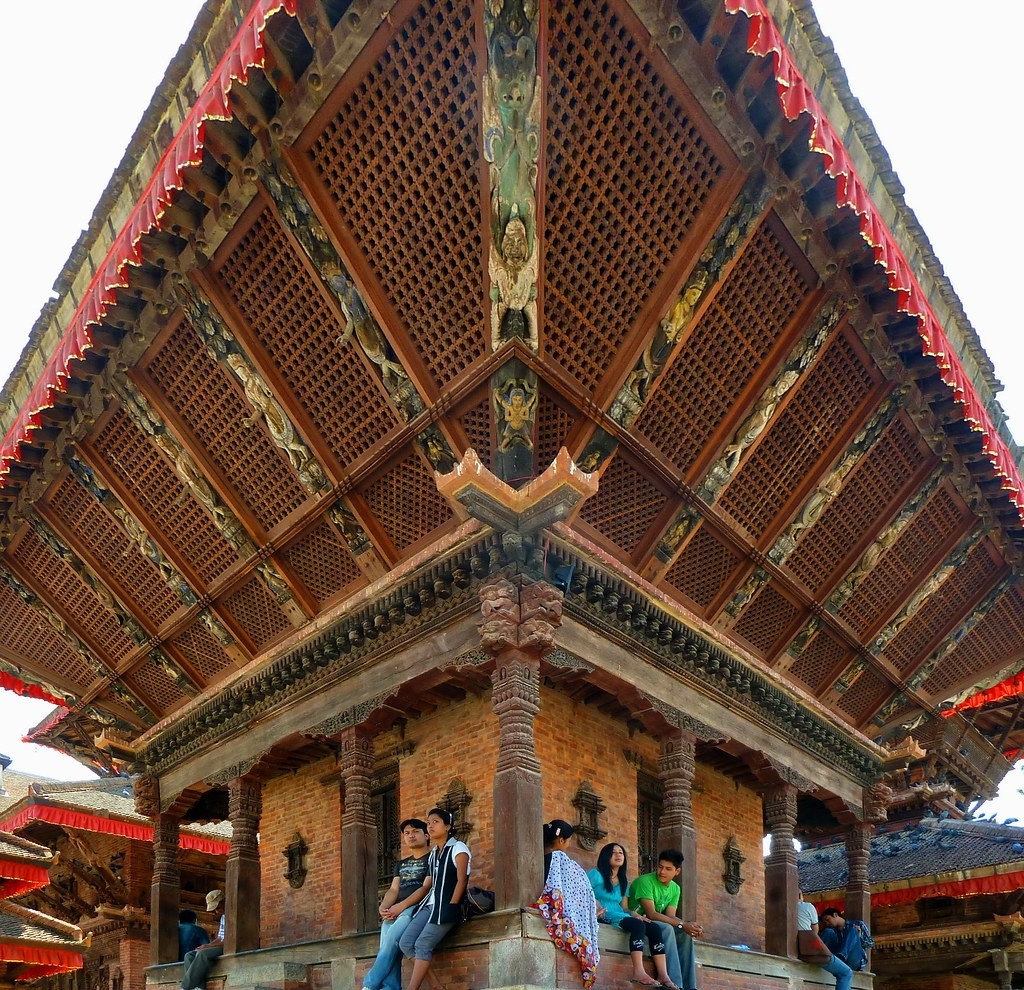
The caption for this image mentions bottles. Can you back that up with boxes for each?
[727,944,751,951]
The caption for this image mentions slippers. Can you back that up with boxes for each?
[662,981,679,990]
[630,977,661,987]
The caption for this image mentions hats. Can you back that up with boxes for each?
[206,889,224,912]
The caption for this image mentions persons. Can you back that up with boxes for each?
[104,495,176,579]
[153,434,228,520]
[892,566,954,632]
[846,512,914,589]
[64,554,113,608]
[363,819,434,990]
[820,908,873,972]
[226,352,311,469]
[317,261,409,387]
[181,890,226,990]
[498,389,535,450]
[178,910,210,962]
[587,843,683,990]
[36,605,93,662]
[787,452,858,541]
[624,848,703,990]
[723,370,800,465]
[626,270,710,400]
[796,889,854,990]
[398,808,472,990]
[543,820,596,923]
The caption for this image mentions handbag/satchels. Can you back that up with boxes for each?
[463,886,495,915]
[799,929,832,963]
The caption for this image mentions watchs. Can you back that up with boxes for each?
[678,920,684,929]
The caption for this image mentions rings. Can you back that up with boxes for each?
[691,933,693,936]
